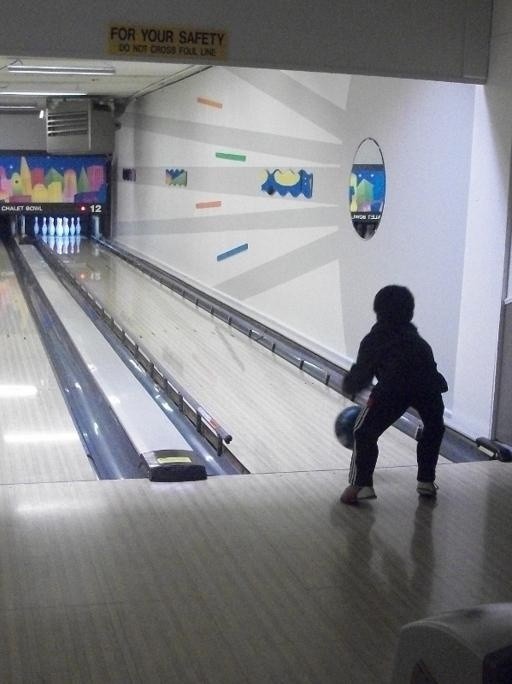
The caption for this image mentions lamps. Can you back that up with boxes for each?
[7,64,115,77]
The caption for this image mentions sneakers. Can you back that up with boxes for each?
[340,485,377,503]
[416,481,436,496]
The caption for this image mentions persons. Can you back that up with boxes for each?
[336,283,448,503]
